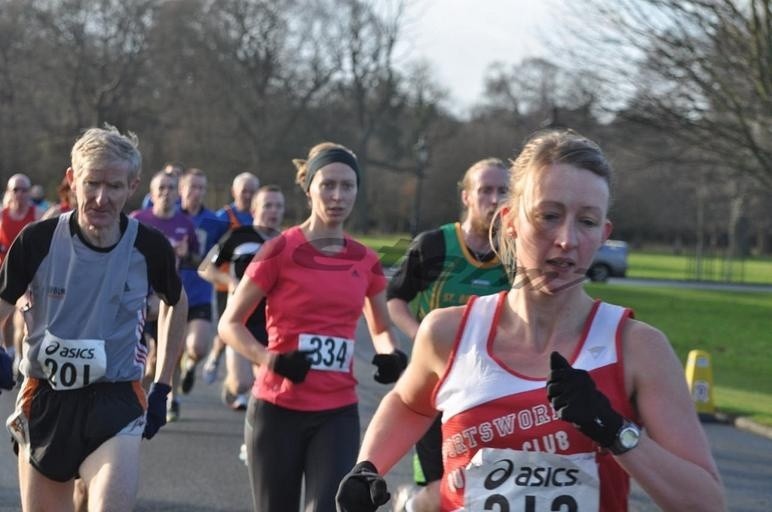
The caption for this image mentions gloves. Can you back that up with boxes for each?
[371,349,408,383]
[274,349,318,384]
[547,352,623,446]
[0,345,16,394]
[143,382,173,440]
[334,461,390,511]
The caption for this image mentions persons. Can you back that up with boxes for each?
[30,183,49,211]
[334,126,724,512]
[1,124,188,512]
[198,186,285,409]
[42,173,76,219]
[202,171,259,383]
[166,168,220,422]
[129,171,198,422]
[141,161,183,210]
[216,143,407,511]
[386,157,509,490]
[2,173,42,387]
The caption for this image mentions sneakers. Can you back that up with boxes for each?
[181,367,194,394]
[202,356,219,384]
[230,393,249,411]
[166,397,179,423]
[221,377,236,406]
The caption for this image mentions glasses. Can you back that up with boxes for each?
[10,186,31,193]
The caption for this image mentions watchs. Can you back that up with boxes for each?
[608,417,641,455]
